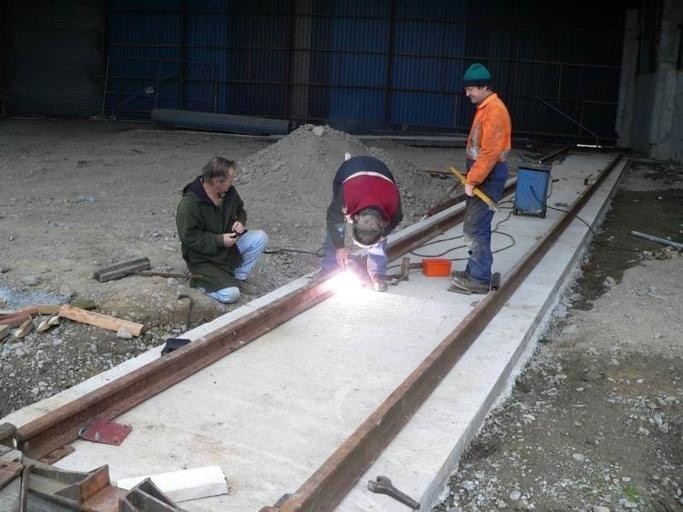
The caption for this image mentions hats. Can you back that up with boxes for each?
[463,64,492,86]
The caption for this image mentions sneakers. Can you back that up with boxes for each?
[449,271,490,293]
[312,263,341,284]
[370,274,387,292]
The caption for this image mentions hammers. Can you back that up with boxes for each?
[95,257,204,282]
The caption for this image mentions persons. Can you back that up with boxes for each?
[171,156,268,306]
[317,154,402,293]
[449,60,513,296]
